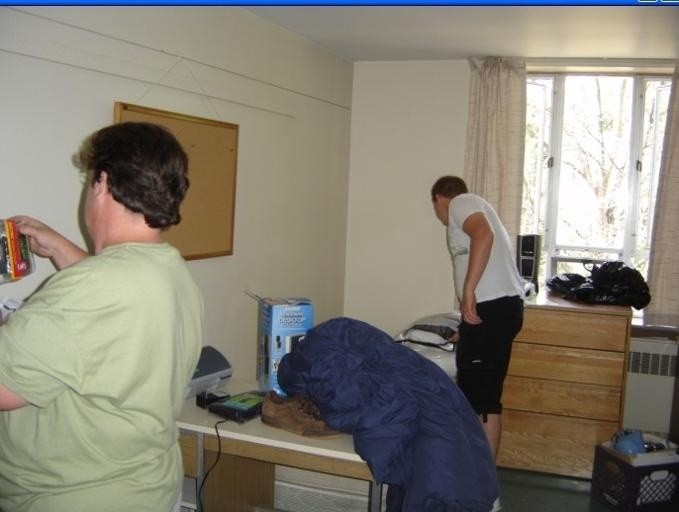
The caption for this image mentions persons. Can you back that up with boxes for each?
[431,175,525,512]
[1,120,206,512]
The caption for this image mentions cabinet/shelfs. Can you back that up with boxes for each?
[492,286,634,482]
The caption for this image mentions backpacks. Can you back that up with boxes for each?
[592,260,652,311]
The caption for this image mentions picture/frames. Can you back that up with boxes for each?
[114,100,239,260]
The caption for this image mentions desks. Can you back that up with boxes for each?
[171,388,385,512]
